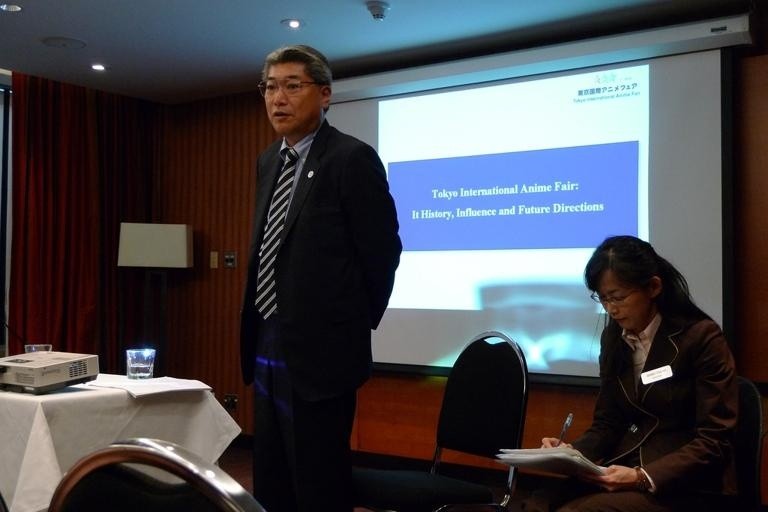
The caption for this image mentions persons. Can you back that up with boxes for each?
[505,236,736,512]
[238,46,403,512]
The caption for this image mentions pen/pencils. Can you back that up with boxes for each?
[556,413,573,447]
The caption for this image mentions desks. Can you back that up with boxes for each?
[1,372,242,511]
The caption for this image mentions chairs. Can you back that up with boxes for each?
[47,438,263,512]
[722,376,763,512]
[346,332,529,512]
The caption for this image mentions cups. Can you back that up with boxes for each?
[125,348,156,380]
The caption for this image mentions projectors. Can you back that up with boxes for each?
[0,350,99,395]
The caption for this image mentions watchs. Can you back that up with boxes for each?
[634,466,645,492]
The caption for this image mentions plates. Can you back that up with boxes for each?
[24,344,52,355]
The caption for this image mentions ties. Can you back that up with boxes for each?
[255,149,299,319]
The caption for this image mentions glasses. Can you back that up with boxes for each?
[258,78,331,97]
[591,280,650,307]
